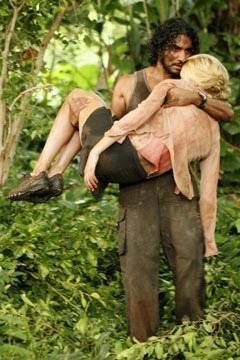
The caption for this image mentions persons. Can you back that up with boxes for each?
[108,17,236,344]
[6,51,232,258]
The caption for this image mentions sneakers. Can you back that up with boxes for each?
[29,174,64,203]
[7,172,50,200]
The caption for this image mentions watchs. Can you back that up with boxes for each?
[197,91,206,109]
